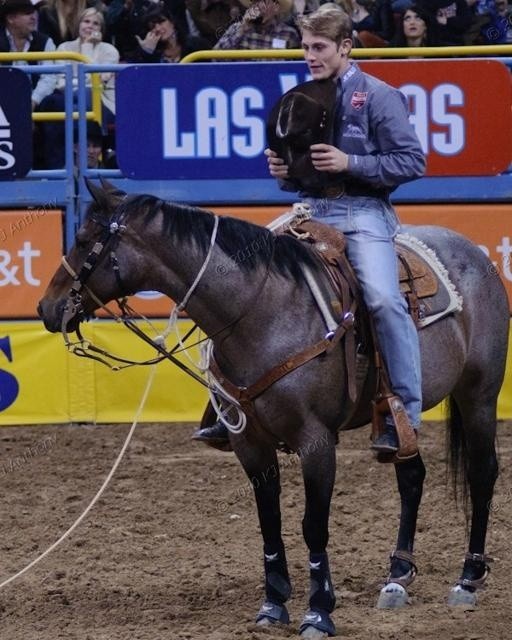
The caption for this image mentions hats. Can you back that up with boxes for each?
[0,0,43,14]
[265,77,339,179]
[238,0,294,15]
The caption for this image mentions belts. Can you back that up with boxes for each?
[298,185,389,201]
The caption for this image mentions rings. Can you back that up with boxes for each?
[94,33,99,36]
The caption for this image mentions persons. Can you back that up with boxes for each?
[35,0,511,68]
[0,1,69,169]
[57,118,108,169]
[189,2,428,454]
[54,5,118,168]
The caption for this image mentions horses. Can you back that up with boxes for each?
[36,174,511,640]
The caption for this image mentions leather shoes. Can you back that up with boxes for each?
[191,416,230,442]
[371,425,417,457]
